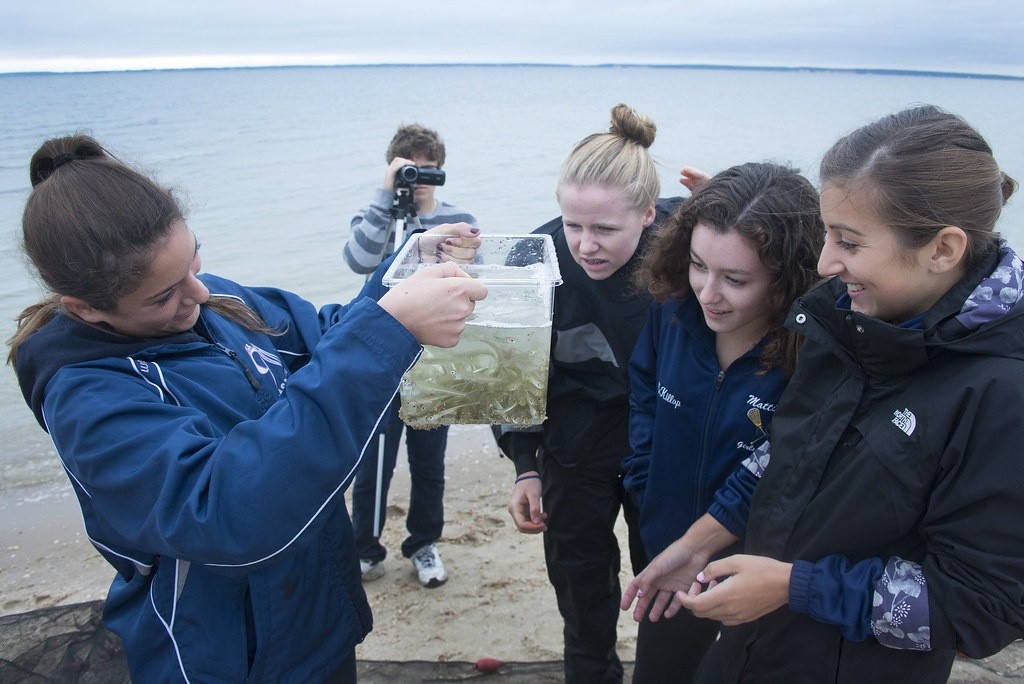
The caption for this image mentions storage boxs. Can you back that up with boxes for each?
[381,231,563,425]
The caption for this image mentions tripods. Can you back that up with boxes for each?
[370,187,424,549]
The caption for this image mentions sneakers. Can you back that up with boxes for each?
[359,558,387,581]
[410,543,449,588]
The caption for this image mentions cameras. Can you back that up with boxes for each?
[394,163,446,188]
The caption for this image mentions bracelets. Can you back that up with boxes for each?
[418,238,420,264]
[515,476,540,484]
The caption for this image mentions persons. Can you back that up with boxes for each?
[490,104,1024,684]
[7,136,490,684]
[343,123,478,585]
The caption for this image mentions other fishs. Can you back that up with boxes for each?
[399,334,550,427]
